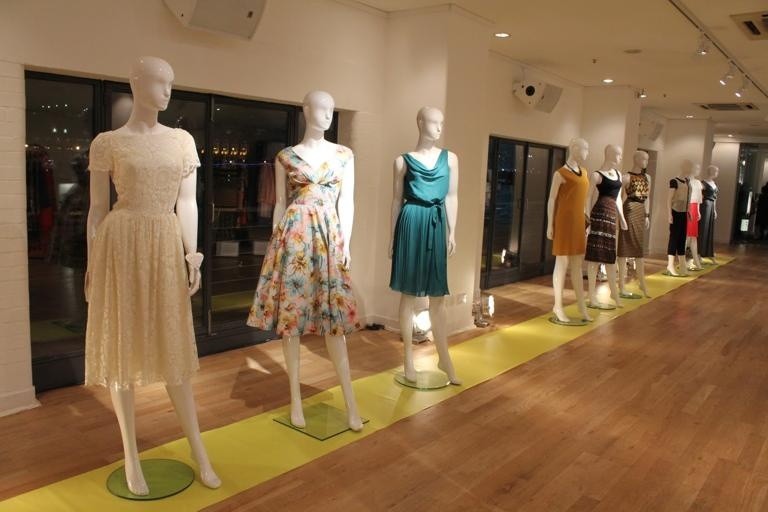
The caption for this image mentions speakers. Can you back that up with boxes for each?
[165,0,267,40]
[513,78,563,114]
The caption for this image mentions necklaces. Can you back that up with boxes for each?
[564,159,583,178]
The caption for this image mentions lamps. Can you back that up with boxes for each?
[719,61,749,98]
[499,246,518,267]
[470,292,496,328]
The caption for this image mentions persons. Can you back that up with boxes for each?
[385,106,466,387]
[585,143,720,309]
[543,138,597,323]
[244,90,364,433]
[80,56,224,497]
